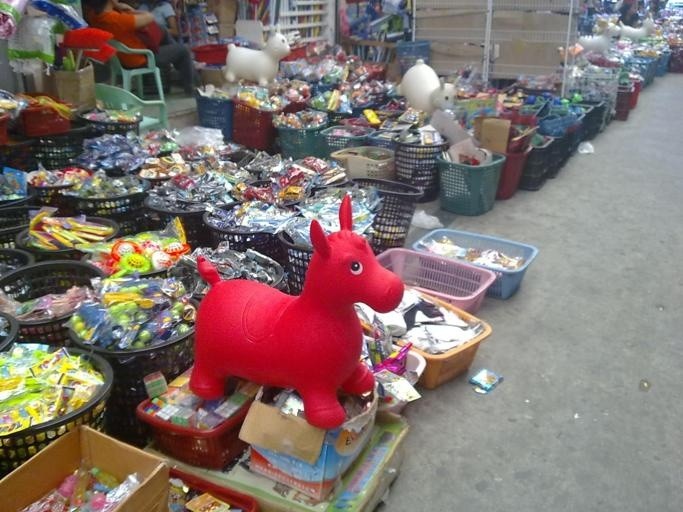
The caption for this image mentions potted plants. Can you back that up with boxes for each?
[43,49,95,116]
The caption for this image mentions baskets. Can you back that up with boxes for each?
[0,85,394,512]
[625,45,670,85]
[492,79,606,190]
[369,130,506,217]
[350,176,539,414]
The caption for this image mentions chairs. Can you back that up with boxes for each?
[87,34,167,141]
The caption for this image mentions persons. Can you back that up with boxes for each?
[137,1,178,46]
[83,0,197,97]
